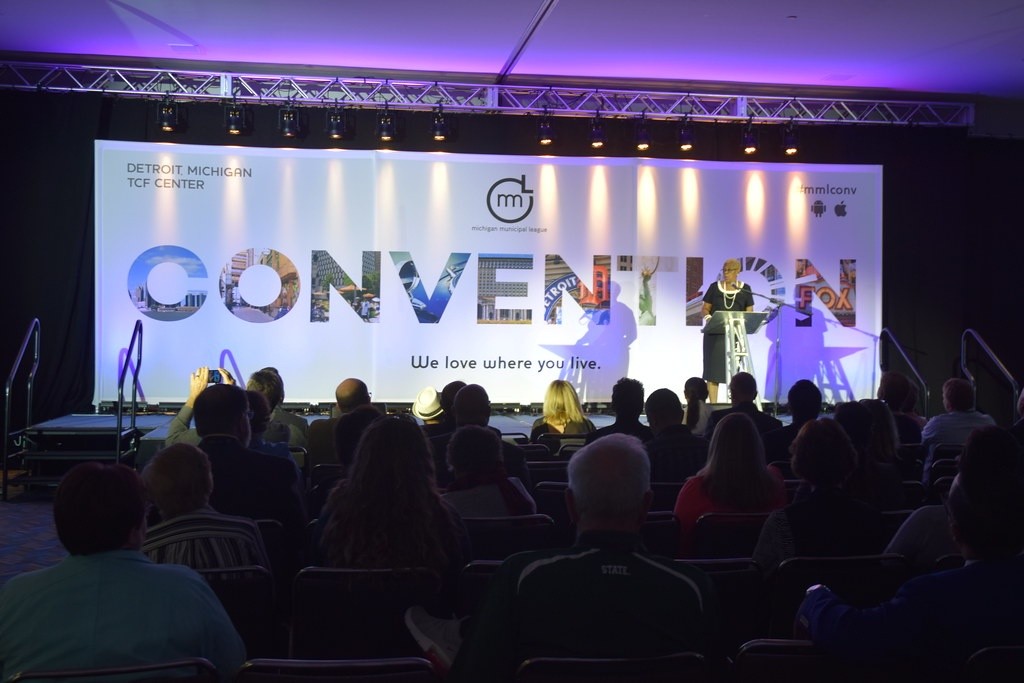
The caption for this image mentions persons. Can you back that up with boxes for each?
[136,442,273,582]
[750,416,887,569]
[142,366,1024,558]
[701,259,755,404]
[0,462,248,683]
[671,410,788,559]
[406,433,703,681]
[789,421,1024,683]
[310,413,470,569]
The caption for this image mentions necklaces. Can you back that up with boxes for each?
[723,281,738,309]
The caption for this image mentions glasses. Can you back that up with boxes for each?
[244,410,254,419]
[720,268,737,275]
[939,491,956,523]
[367,393,372,398]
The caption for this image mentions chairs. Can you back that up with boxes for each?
[11,433,1024,683]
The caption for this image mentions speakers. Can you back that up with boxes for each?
[329,403,387,419]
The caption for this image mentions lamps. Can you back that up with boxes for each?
[592,110,604,150]
[784,115,797,154]
[678,112,693,153]
[326,98,346,139]
[434,103,444,139]
[743,115,757,153]
[155,95,178,132]
[278,102,301,136]
[636,109,650,152]
[376,101,396,139]
[540,105,552,145]
[223,98,246,134]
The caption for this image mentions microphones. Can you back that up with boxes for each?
[731,282,739,291]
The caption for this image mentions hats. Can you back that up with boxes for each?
[411,386,444,420]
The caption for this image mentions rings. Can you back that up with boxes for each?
[196,375,199,377]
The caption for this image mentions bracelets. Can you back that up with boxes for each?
[704,315,711,319]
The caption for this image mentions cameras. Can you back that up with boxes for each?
[206,369,222,384]
